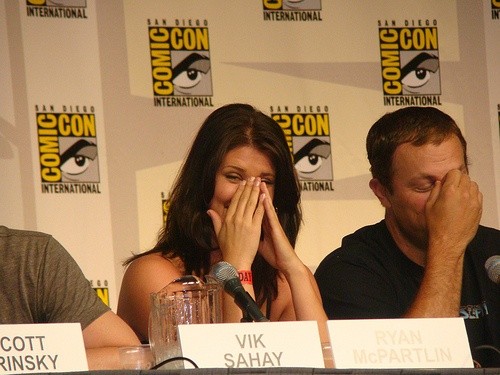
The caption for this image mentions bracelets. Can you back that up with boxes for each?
[237,269,253,285]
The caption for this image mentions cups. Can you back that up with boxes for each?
[120,344,153,370]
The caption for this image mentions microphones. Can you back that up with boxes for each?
[208,261,269,322]
[485,255,500,285]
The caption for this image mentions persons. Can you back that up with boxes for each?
[313,106,500,367]
[0,224,150,371]
[116,104,330,345]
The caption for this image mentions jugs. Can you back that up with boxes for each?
[147,274,225,371]
